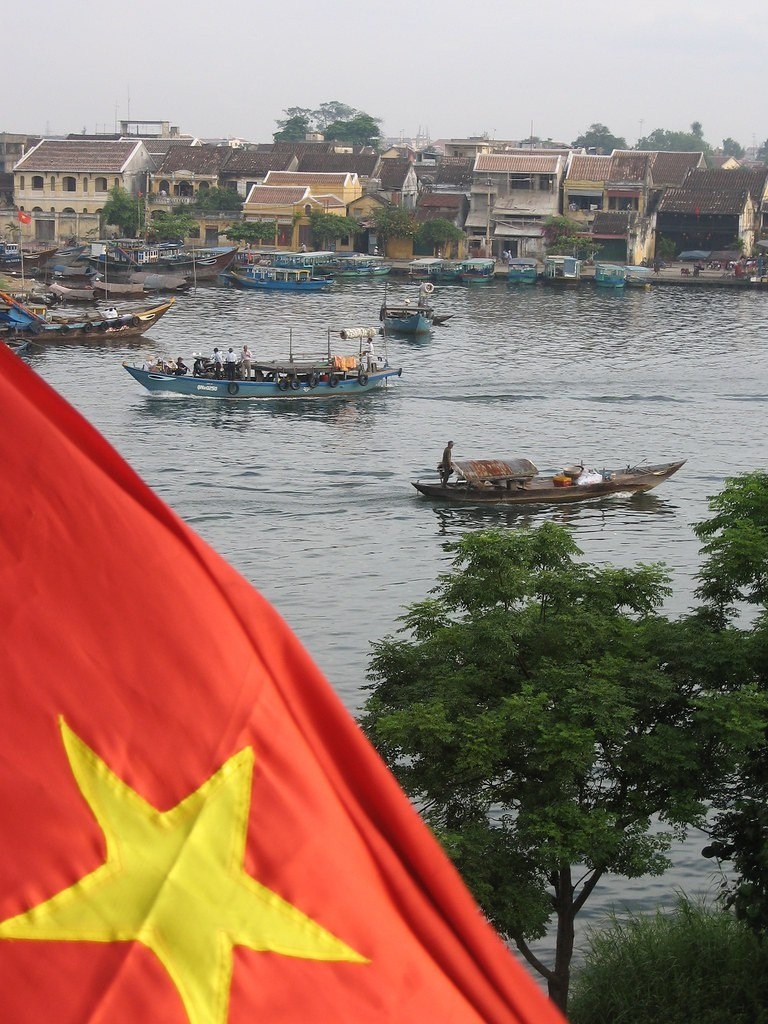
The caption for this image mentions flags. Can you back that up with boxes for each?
[0,335,566,1024]
[18,210,31,225]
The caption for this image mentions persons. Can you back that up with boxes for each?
[240,345,252,381]
[503,249,511,263]
[161,188,167,196]
[694,257,756,271]
[145,355,178,375]
[625,201,632,211]
[568,200,577,211]
[211,348,222,380]
[226,348,238,380]
[362,337,374,372]
[374,246,378,256]
[102,307,118,318]
[176,357,190,375]
[31,288,36,296]
[297,242,306,253]
[442,441,454,487]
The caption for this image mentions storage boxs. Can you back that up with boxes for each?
[553,475,571,487]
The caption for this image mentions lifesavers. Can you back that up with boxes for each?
[227,382,240,395]
[308,372,320,388]
[85,323,93,332]
[101,322,109,331]
[424,282,435,293]
[330,375,339,388]
[133,317,141,327]
[115,320,123,329]
[60,325,69,336]
[359,374,370,386]
[291,379,301,390]
[279,379,289,391]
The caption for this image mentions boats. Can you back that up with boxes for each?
[380,281,436,339]
[410,455,689,505]
[0,288,174,341]
[391,312,455,323]
[507,258,539,286]
[440,262,462,282]
[229,264,334,290]
[623,265,650,287]
[462,257,496,283]
[121,327,403,399]
[594,263,626,289]
[408,258,444,280]
[0,189,392,310]
[542,255,583,286]
